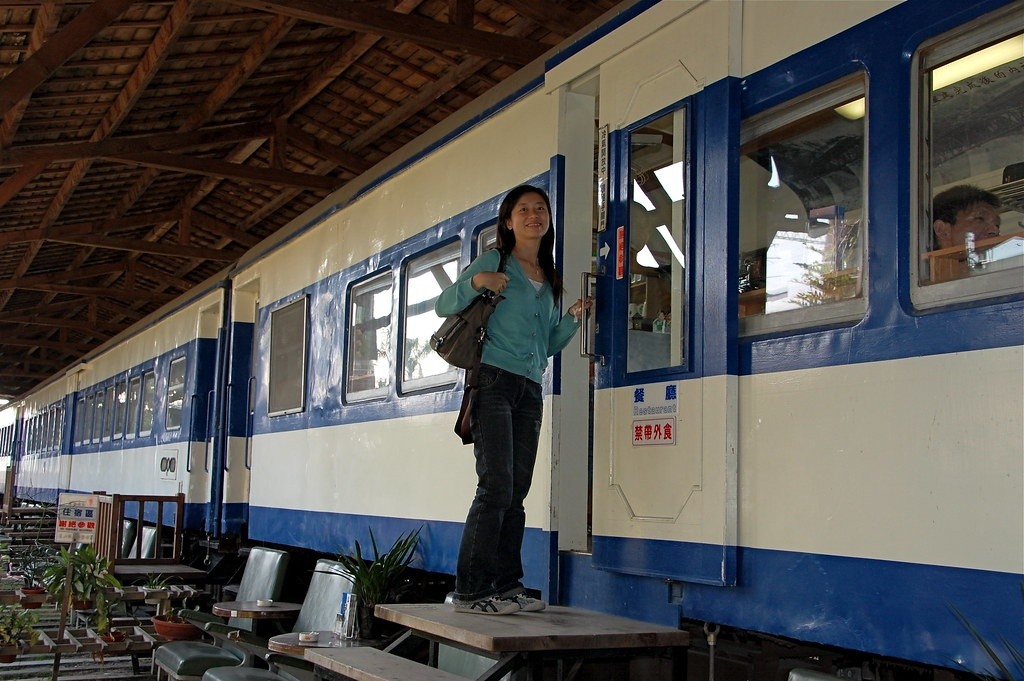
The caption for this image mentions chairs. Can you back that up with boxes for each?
[202,560,361,681]
[154,549,289,681]
[67,520,157,558]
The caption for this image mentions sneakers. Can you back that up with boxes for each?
[455,597,519,614]
[489,594,546,610]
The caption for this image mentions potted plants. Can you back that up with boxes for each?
[0,606,40,663]
[307,522,424,640]
[151,597,210,639]
[130,570,184,600]
[11,544,58,608]
[43,544,123,616]
[97,618,126,643]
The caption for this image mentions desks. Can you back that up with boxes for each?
[266,630,385,655]
[212,601,302,636]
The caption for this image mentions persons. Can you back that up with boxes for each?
[932,184,1002,277]
[435,185,593,614]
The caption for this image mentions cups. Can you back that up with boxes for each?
[629,302,646,317]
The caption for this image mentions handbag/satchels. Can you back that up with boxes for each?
[430,247,506,371]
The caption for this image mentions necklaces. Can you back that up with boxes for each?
[534,264,539,275]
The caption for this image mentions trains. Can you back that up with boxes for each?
[0,0,1024,681]
